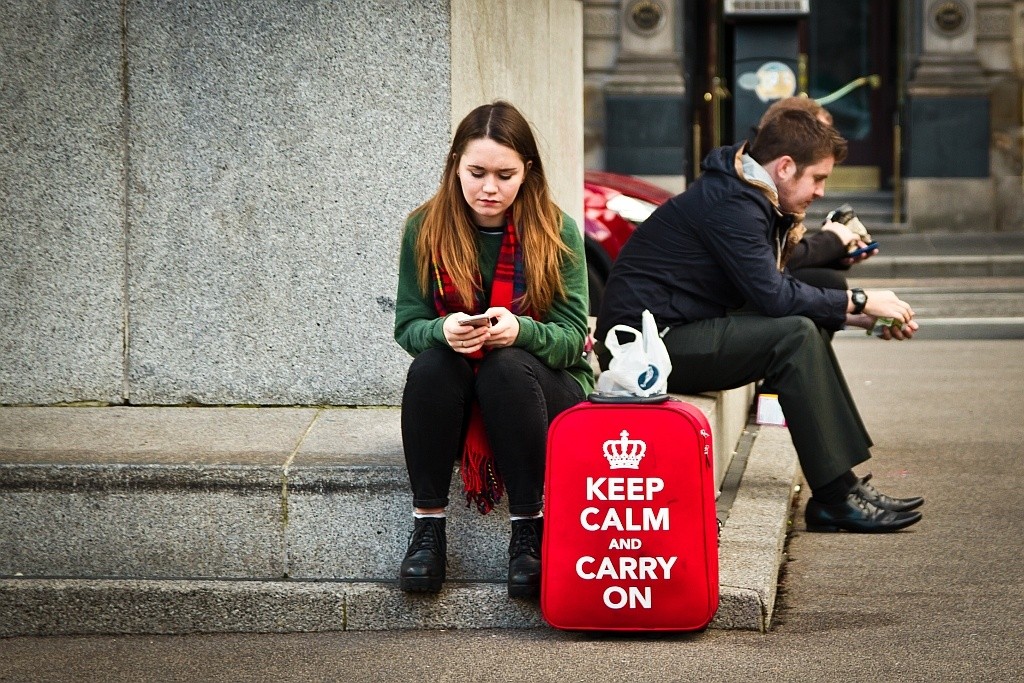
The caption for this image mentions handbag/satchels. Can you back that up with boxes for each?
[594,308,672,398]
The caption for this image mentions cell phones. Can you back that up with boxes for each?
[457,314,492,329]
[847,241,880,257]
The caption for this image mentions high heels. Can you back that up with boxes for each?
[508,517,543,595]
[400,516,447,593]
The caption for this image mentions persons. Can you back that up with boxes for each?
[394,101,595,598]
[595,97,923,533]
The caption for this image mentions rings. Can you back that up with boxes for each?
[461,340,463,346]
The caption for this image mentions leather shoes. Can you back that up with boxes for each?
[850,475,924,511]
[804,493,922,533]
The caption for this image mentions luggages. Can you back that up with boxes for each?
[538,390,720,632]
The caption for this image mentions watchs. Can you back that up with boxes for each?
[850,288,867,314]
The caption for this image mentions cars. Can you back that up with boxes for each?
[583,168,677,317]
